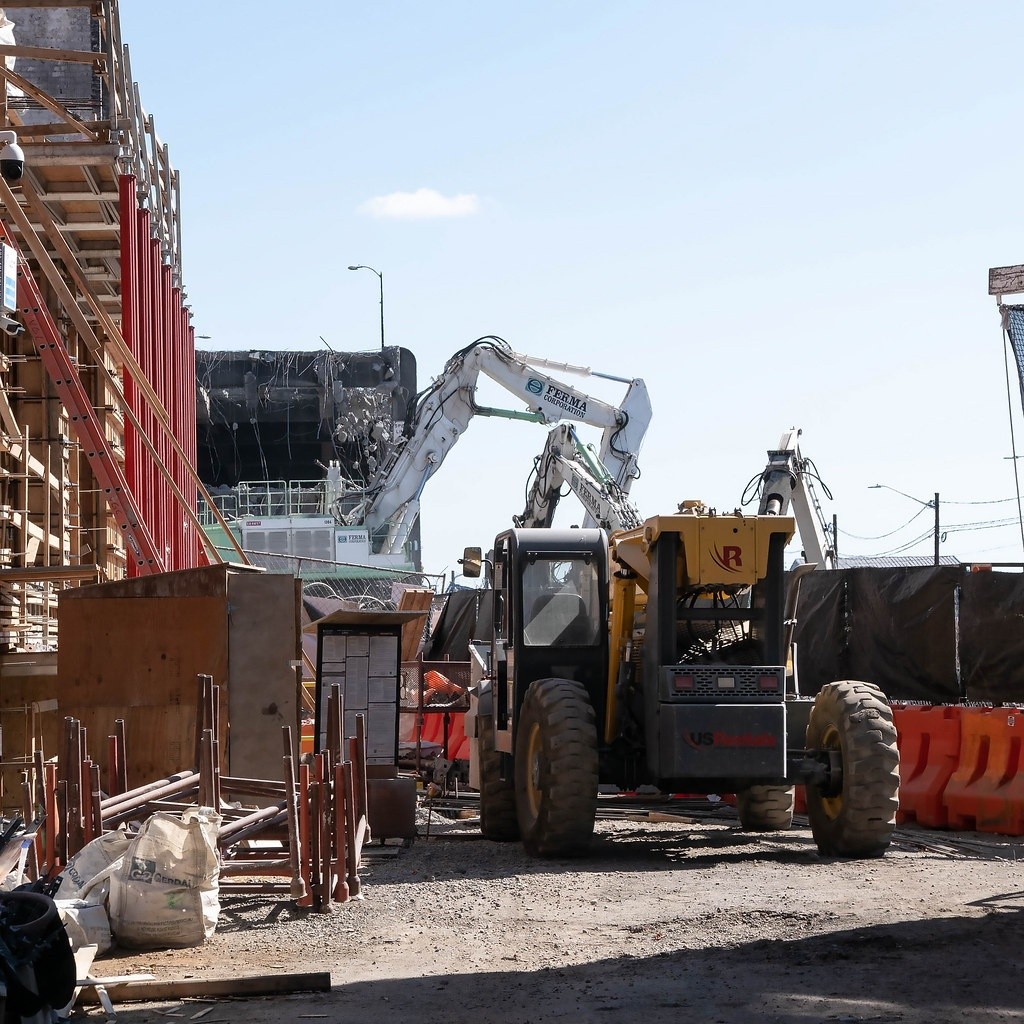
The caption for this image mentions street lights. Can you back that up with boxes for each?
[348,265,386,353]
[867,485,940,565]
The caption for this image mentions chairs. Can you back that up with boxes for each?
[529,593,589,643]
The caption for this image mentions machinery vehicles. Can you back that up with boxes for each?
[329,334,902,861]
